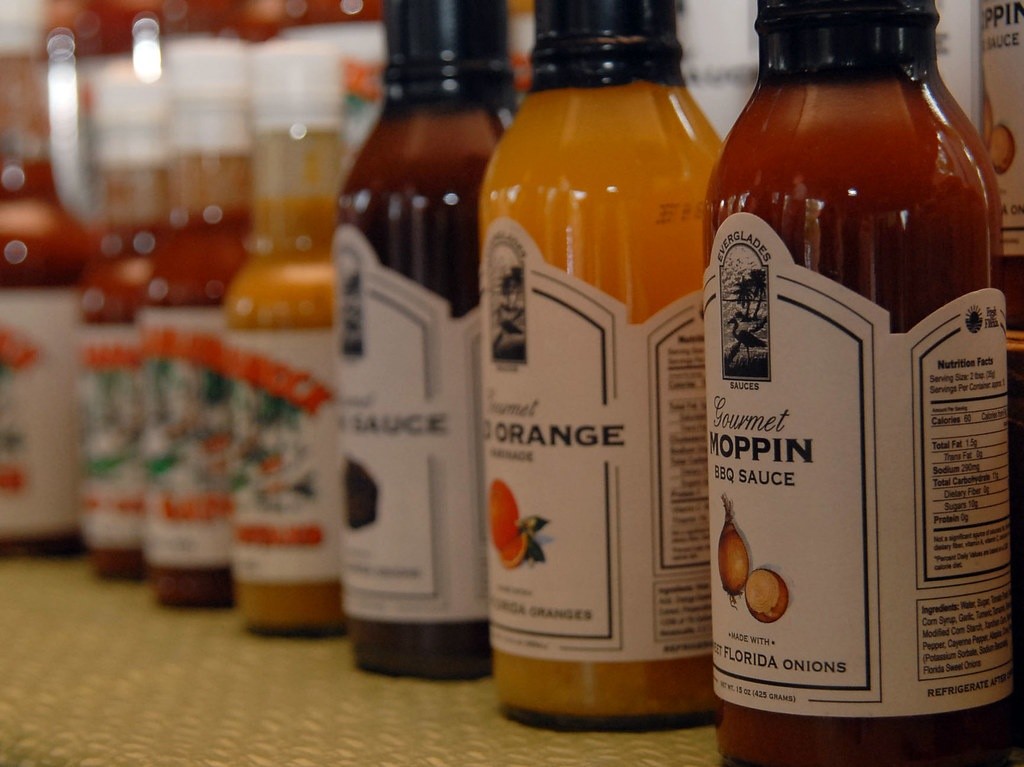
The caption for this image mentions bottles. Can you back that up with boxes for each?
[698,0,1009,767]
[140,36,257,610]
[481,0,727,730]
[223,40,340,640]
[334,0,522,682]
[72,61,170,578]
[278,0,385,145]
[0,39,70,558]
